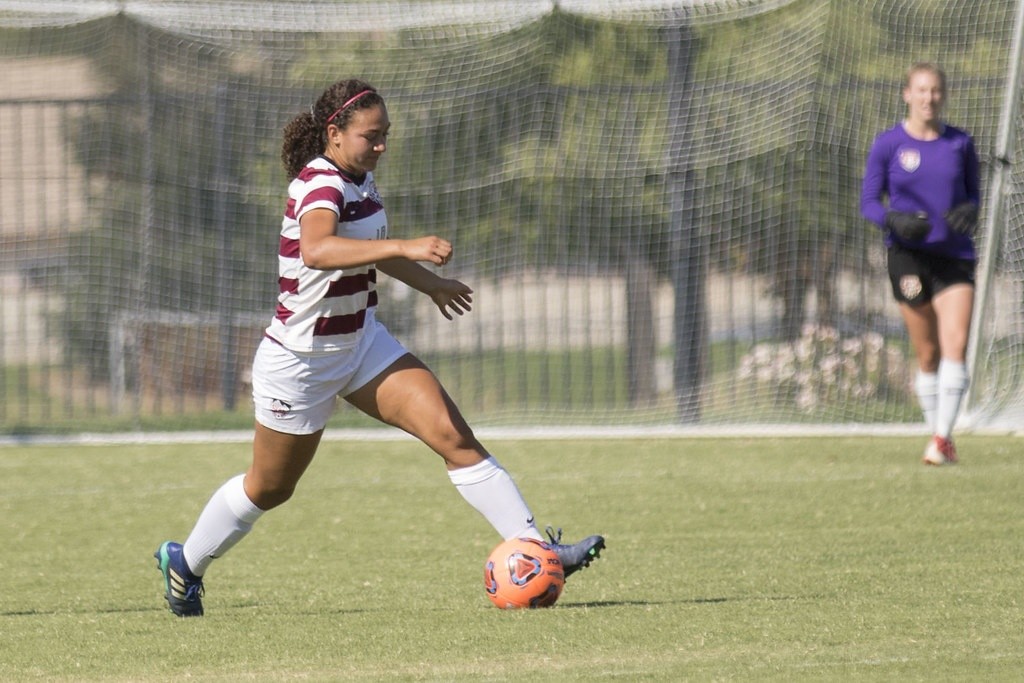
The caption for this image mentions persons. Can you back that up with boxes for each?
[860,64,981,467]
[155,79,606,617]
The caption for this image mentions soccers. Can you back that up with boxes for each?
[484,536,565,610]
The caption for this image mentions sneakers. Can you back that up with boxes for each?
[542,525,606,584]
[154,541,205,618]
[922,434,958,466]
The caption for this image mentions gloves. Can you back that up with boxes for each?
[942,202,981,238]
[886,209,933,244]
